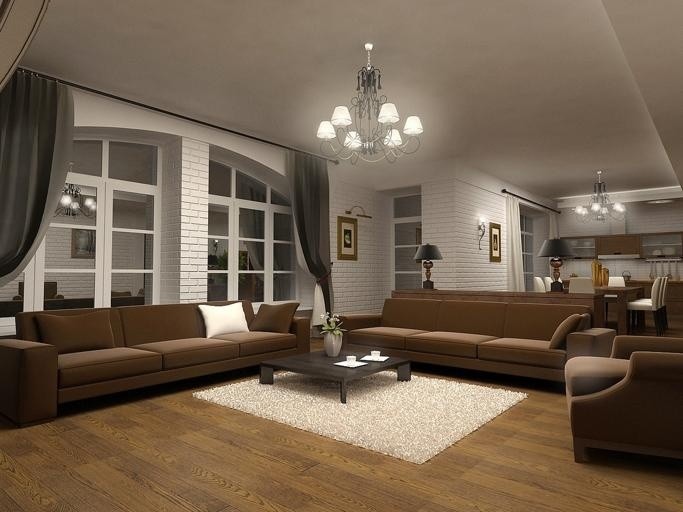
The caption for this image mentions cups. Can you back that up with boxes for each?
[370,350,383,357]
[346,354,357,364]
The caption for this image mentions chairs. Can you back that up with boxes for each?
[561,332,681,467]
[529,271,671,337]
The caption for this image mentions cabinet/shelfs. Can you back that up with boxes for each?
[558,234,598,260]
[642,281,682,330]
[640,231,683,259]
[597,234,639,257]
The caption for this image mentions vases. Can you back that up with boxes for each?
[322,329,343,358]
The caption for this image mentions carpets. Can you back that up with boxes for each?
[190,367,530,466]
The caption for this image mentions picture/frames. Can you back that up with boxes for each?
[334,214,360,262]
[487,221,503,263]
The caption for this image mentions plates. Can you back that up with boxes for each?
[651,247,676,256]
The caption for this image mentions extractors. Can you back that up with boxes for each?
[597,254,640,261]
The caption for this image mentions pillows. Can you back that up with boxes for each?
[194,296,251,341]
[248,300,299,335]
[547,309,588,350]
[30,308,117,355]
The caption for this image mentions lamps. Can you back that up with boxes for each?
[569,169,628,224]
[534,238,578,297]
[344,205,373,219]
[475,213,488,251]
[49,179,101,218]
[313,40,426,166]
[413,242,442,290]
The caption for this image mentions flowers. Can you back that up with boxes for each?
[317,309,347,336]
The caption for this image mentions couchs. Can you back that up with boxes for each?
[0,280,146,319]
[0,297,312,425]
[330,297,615,390]
